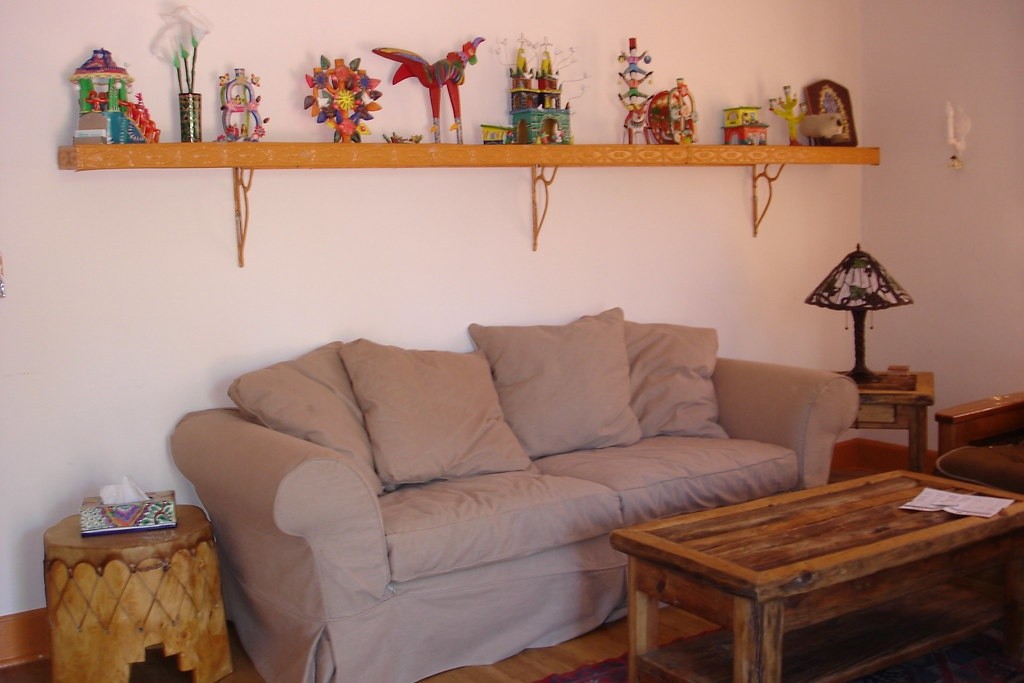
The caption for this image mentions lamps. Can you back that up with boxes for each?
[942,99,972,171]
[804,243,915,384]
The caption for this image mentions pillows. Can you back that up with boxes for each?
[338,337,531,483]
[227,340,384,497]
[580,315,728,440]
[468,306,643,457]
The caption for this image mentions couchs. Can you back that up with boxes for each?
[170,355,858,683]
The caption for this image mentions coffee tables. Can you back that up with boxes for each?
[610,469,1024,683]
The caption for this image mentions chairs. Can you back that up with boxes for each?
[935,391,1024,495]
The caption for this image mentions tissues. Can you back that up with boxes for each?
[80,474,178,538]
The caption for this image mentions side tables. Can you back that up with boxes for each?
[42,504,232,683]
[834,371,936,472]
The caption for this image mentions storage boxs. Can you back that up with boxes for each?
[720,105,770,146]
